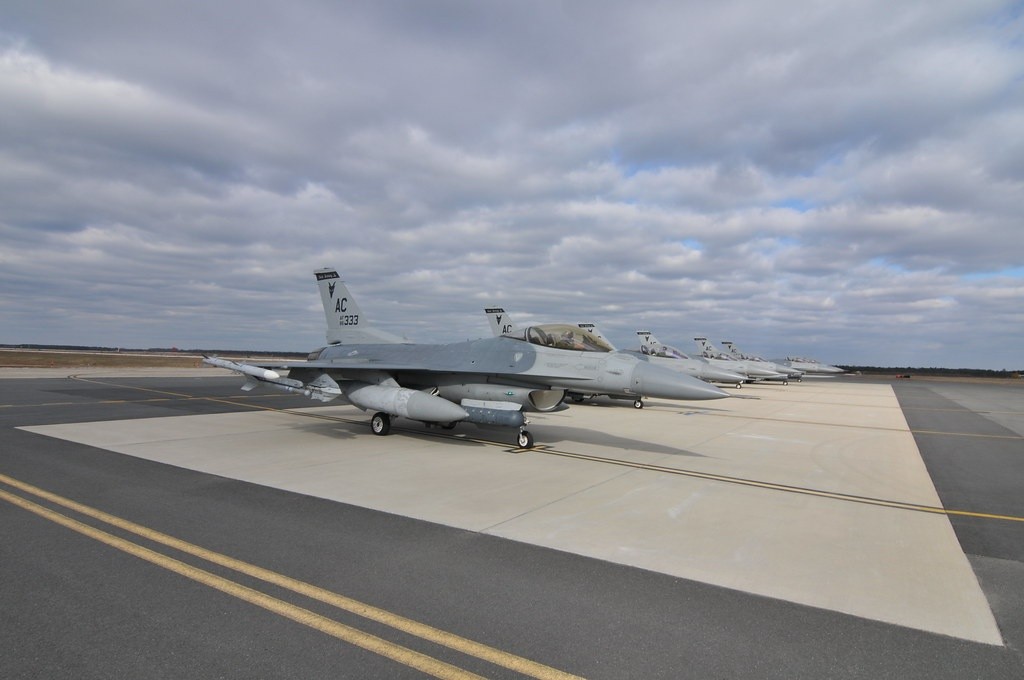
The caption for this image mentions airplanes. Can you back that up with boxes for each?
[476,304,851,411]
[197,268,732,451]
